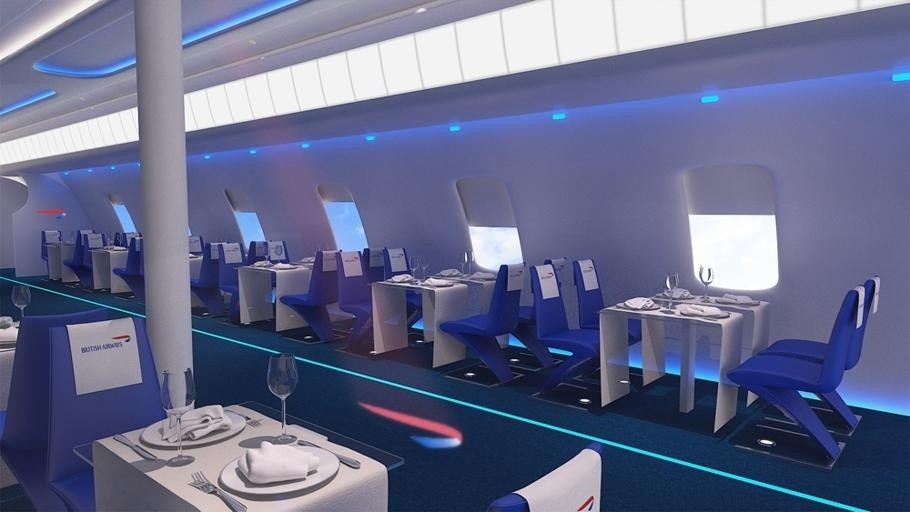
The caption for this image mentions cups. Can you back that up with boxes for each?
[699,264,714,304]
[117,235,122,246]
[664,272,679,314]
[464,252,474,276]
[266,352,298,445]
[263,247,270,261]
[11,285,31,328]
[160,367,196,467]
[106,235,112,250]
[417,257,428,283]
[409,256,417,278]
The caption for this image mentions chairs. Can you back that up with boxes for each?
[336,251,372,348]
[189,235,204,254]
[383,248,423,333]
[488,440,604,510]
[247,241,269,264]
[64,229,94,288]
[758,272,880,429]
[727,285,868,460]
[45,316,167,512]
[573,259,642,346]
[113,237,143,298]
[364,248,385,286]
[84,233,105,269]
[511,257,567,367]
[41,230,62,271]
[191,242,228,297]
[217,243,246,322]
[280,250,343,343]
[439,262,527,381]
[530,264,600,394]
[123,232,143,248]
[267,240,290,263]
[0,306,111,512]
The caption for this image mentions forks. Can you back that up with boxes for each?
[191,471,248,511]
[223,409,262,427]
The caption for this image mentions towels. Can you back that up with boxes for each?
[392,273,412,283]
[470,271,495,281]
[664,287,692,299]
[237,440,319,484]
[0,325,18,342]
[113,246,125,250]
[254,260,271,266]
[161,405,231,443]
[440,269,462,277]
[623,296,654,310]
[302,257,315,262]
[274,263,291,268]
[424,277,448,286]
[680,304,721,316]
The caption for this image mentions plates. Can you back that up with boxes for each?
[114,249,127,251]
[655,293,694,300]
[426,283,454,286]
[219,445,340,495]
[251,264,269,268]
[436,273,462,277]
[271,266,297,269]
[470,276,493,281]
[680,310,731,319]
[716,299,751,306]
[616,303,660,311]
[140,411,247,449]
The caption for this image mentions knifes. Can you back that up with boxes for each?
[113,433,156,460]
[296,439,361,469]
